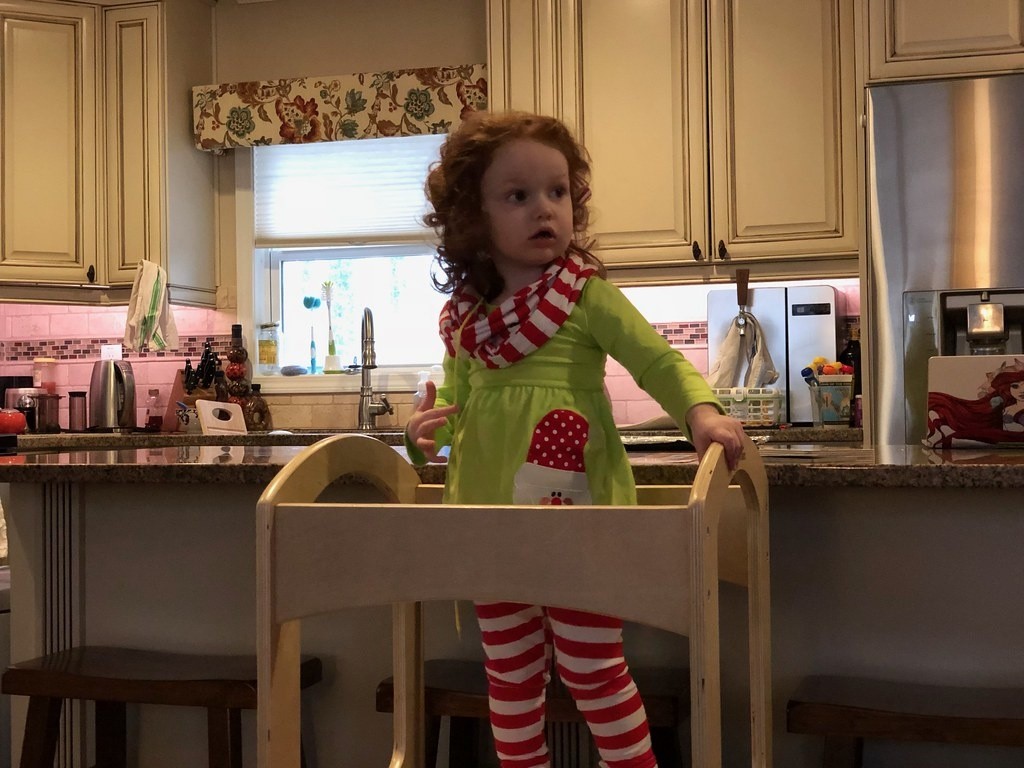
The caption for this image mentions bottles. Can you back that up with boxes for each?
[227,324,253,387]
[145,389,163,431]
[257,323,286,376]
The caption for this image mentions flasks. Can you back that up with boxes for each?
[67,392,87,431]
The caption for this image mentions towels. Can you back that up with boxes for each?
[122,258,180,352]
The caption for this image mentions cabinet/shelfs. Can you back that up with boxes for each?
[557,0,864,291]
[866,0,1024,87]
[0,0,217,308]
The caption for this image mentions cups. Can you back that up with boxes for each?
[33,357,58,395]
[809,385,822,429]
[15,407,36,432]
[818,375,852,429]
[38,394,59,433]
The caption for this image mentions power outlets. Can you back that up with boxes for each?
[99,344,123,361]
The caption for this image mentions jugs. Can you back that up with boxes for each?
[89,360,138,433]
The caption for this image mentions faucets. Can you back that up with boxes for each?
[355,308,395,435]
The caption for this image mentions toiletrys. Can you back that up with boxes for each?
[413,371,431,415]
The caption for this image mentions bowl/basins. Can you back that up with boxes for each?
[176,408,220,435]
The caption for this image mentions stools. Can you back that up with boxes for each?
[0,647,321,768]
[785,674,1024,768]
[375,660,740,768]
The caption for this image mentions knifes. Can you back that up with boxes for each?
[181,341,217,395]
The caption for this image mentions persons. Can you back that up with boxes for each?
[403,108,746,768]
